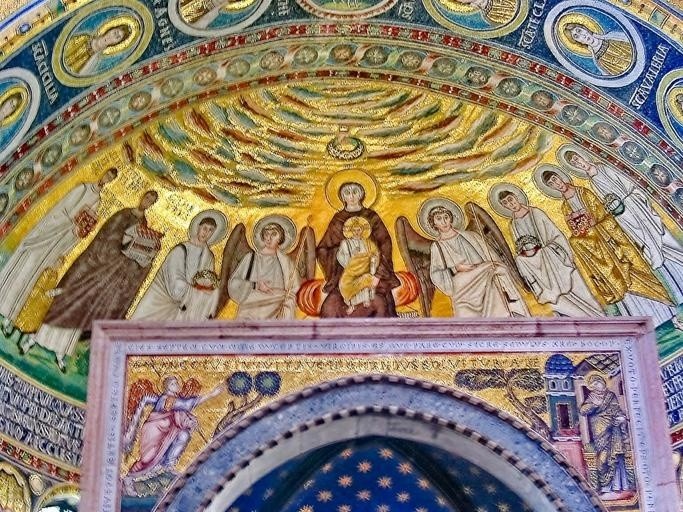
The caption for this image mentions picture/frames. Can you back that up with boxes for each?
[79,318,683,509]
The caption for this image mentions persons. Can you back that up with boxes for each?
[0,92,23,125]
[126,377,225,479]
[449,0,517,27]
[64,22,132,75]
[579,375,630,495]
[177,1,242,29]
[674,93,683,116]
[562,23,633,78]
